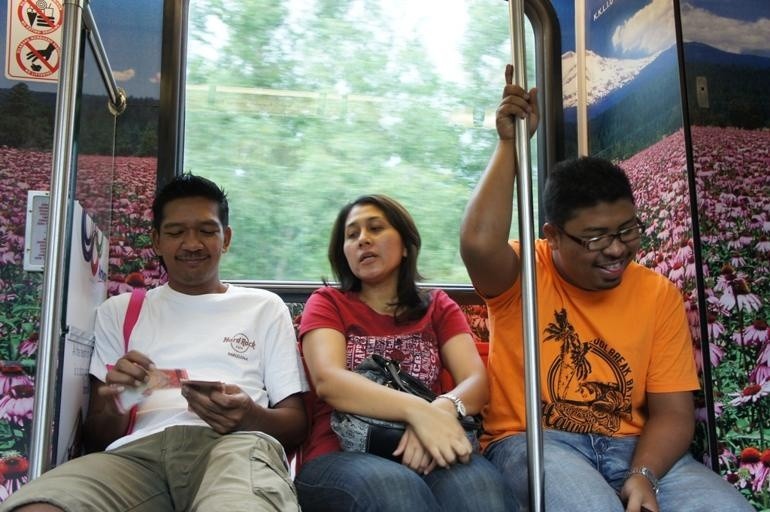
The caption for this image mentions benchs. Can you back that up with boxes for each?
[126,339,493,488]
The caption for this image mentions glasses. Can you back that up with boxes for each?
[556,216,646,252]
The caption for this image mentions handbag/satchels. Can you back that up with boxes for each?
[330,353,482,465]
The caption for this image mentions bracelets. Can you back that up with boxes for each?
[626,466,662,495]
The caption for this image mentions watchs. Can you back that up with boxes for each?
[438,394,467,420]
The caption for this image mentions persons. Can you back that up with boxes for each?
[2,169,313,511]
[295,193,521,510]
[457,63,755,511]
